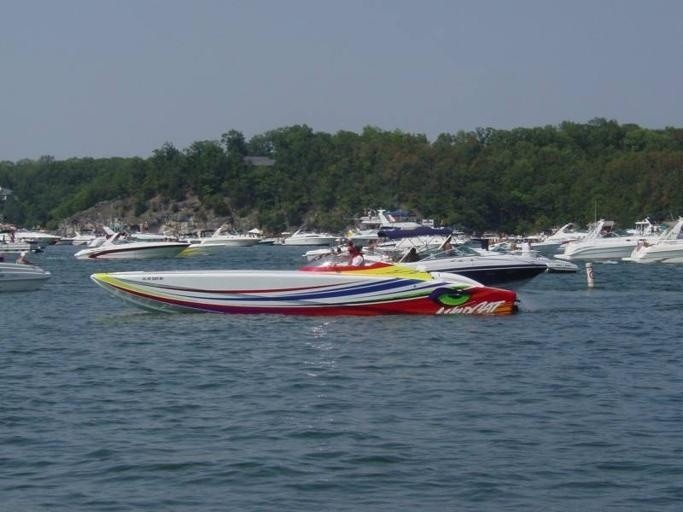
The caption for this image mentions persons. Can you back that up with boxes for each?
[346,239,364,267]
[404,248,418,263]
[443,236,451,252]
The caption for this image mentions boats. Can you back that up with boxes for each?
[57,200,682,274]
[300,234,548,285]
[72,231,191,261]
[89,264,520,317]
[0,253,52,292]
[186,220,263,249]
[0,230,61,250]
